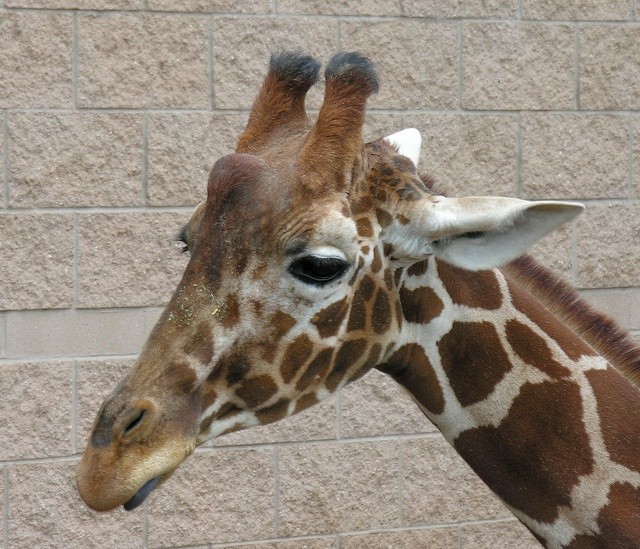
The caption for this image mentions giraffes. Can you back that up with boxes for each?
[77,48,639,549]
